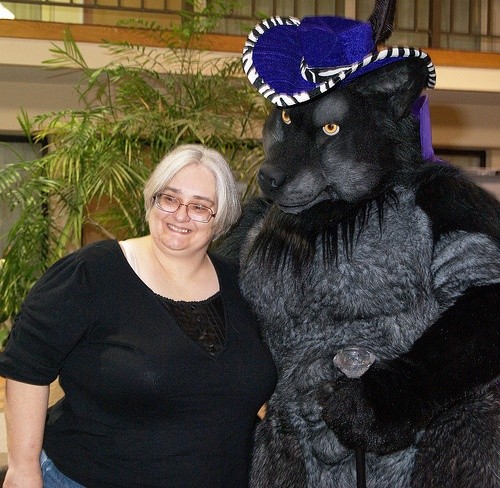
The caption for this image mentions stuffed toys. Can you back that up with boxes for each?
[206,14,500,488]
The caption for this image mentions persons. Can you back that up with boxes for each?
[0,143,279,487]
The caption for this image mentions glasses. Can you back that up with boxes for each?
[152,191,217,223]
